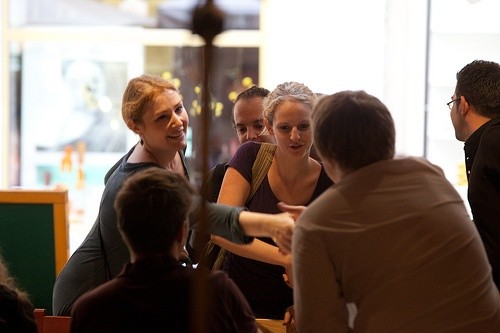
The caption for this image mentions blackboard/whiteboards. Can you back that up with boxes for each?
[0,189,69,316]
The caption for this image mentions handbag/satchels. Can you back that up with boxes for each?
[198,240,227,274]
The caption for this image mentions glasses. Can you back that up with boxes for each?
[447,96,461,110]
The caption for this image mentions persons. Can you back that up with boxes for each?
[67,166,260,333]
[446,59,500,290]
[184,87,271,270]
[209,80,336,322]
[0,284,37,333]
[52,74,296,318]
[291,89,500,333]
